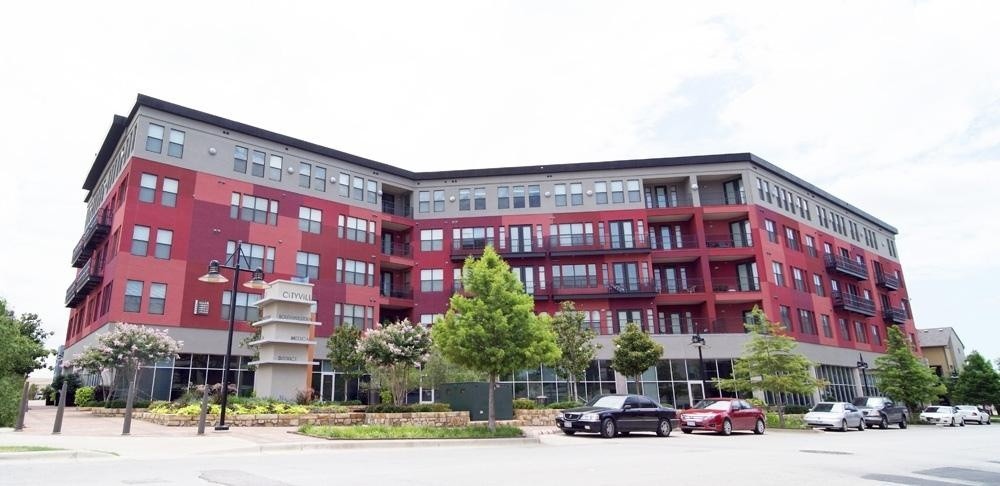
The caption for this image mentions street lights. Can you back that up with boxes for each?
[951,372,959,383]
[198,240,272,430]
[857,352,869,396]
[690,328,708,398]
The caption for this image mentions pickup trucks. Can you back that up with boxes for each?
[851,396,911,428]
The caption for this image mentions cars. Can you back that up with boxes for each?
[555,394,765,438]
[33,391,43,399]
[803,402,865,432]
[920,405,990,426]
